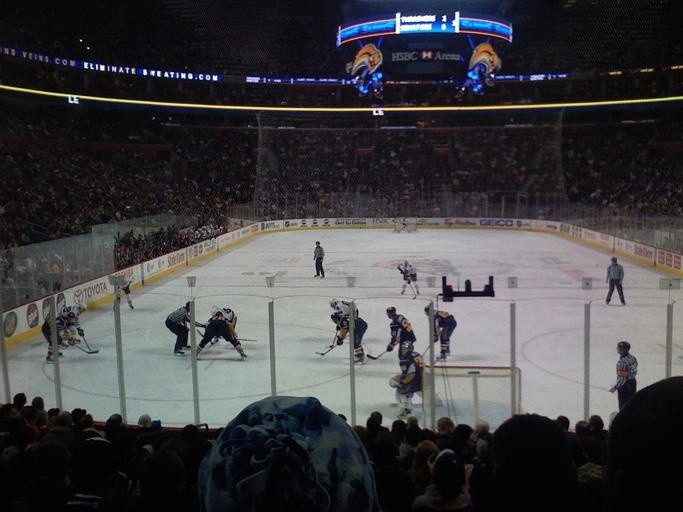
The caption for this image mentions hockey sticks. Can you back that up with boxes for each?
[316,344,338,356]
[330,330,338,348]
[410,283,416,300]
[196,328,219,345]
[76,344,99,354]
[367,350,388,360]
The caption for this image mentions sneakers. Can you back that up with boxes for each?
[46,342,69,361]
[354,358,362,362]
[436,353,446,359]
[182,345,191,348]
[174,349,184,354]
[241,353,247,358]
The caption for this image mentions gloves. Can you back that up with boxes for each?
[337,339,343,345]
[387,342,395,352]
[77,328,84,336]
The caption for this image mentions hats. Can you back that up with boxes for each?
[71,408,86,418]
[197,395,380,512]
[47,408,59,417]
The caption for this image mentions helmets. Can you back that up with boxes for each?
[67,311,76,319]
[617,342,631,352]
[79,302,87,311]
[386,307,396,312]
[401,340,413,356]
[329,298,338,308]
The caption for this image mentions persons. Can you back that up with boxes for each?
[164,301,248,362]
[604,257,626,306]
[261,76,682,253]
[329,299,369,366]
[1,372,682,512]
[385,307,425,418]
[609,341,639,410]
[314,241,326,280]
[1,82,261,311]
[397,260,420,295]
[41,302,88,365]
[423,303,457,362]
